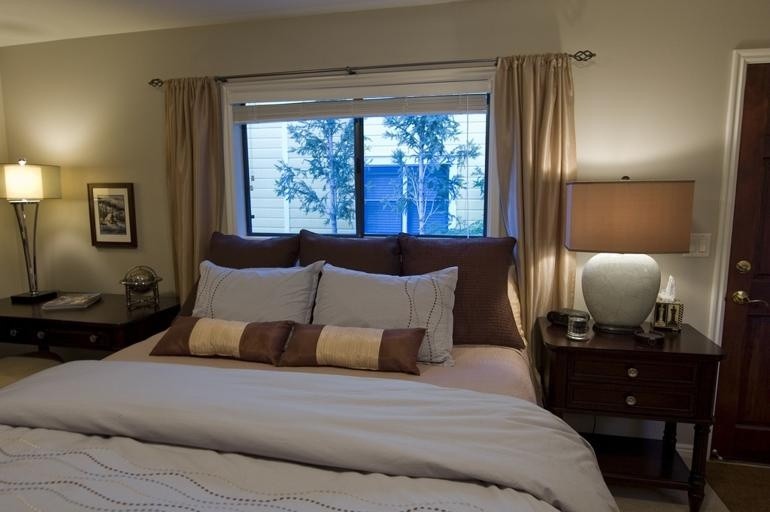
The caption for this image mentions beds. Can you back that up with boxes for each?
[0,316,619,512]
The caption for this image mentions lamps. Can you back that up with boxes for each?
[564,176,695,336]
[0,158,62,305]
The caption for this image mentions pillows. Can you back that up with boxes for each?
[180,231,299,317]
[276,323,427,376]
[398,233,525,349]
[311,262,458,368]
[148,314,294,365]
[191,260,326,326]
[299,229,400,275]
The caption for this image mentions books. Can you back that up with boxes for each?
[42,293,101,309]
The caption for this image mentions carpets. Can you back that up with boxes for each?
[704,463,770,512]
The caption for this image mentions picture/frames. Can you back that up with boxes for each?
[88,183,138,248]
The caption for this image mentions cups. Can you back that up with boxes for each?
[567,311,595,340]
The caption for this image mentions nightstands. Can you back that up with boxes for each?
[0,291,180,361]
[535,315,727,512]
